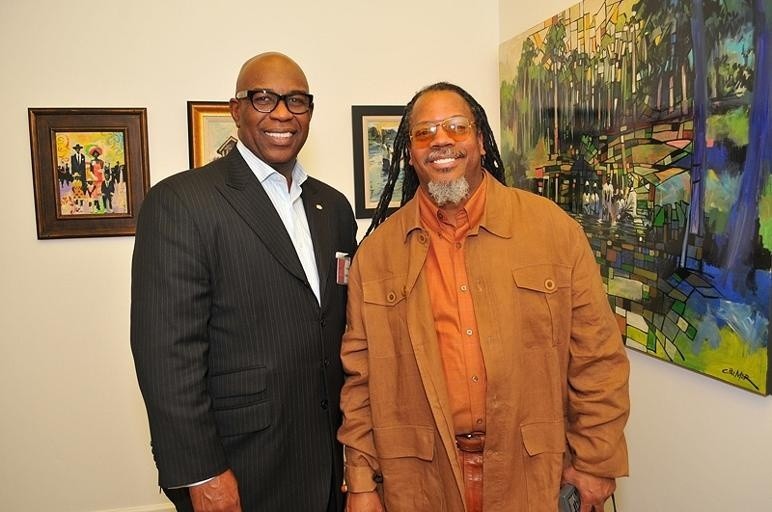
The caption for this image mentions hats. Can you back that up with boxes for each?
[72,144,83,149]
[89,147,102,155]
[71,172,81,177]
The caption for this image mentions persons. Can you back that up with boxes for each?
[57,143,127,214]
[336,80,630,512]
[130,52,358,512]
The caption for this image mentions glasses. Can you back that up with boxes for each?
[235,89,313,115]
[408,115,477,149]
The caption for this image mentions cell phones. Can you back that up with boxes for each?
[559,484,581,512]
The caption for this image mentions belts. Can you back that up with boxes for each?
[455,431,486,452]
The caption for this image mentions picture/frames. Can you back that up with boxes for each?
[186,100,238,170]
[349,105,407,220]
[29,108,151,240]
